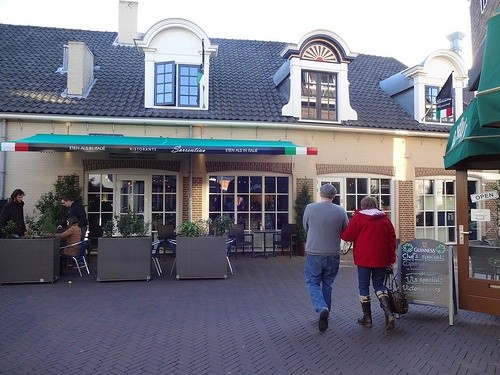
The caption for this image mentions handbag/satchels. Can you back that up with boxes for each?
[380,268,409,314]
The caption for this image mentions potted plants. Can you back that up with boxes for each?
[294,185,311,257]
[97,211,152,282]
[175,213,231,280]
[0,208,63,284]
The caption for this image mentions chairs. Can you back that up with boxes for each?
[229,224,254,260]
[273,223,299,258]
[151,224,176,277]
[60,239,91,277]
[226,238,236,275]
[88,227,103,250]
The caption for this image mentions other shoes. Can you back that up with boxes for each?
[318,308,329,332]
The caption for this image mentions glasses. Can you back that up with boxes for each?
[62,201,67,204]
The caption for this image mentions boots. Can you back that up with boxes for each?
[358,295,372,328]
[375,290,396,333]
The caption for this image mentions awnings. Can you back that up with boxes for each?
[476,7,500,128]
[443,97,500,170]
[0,133,319,156]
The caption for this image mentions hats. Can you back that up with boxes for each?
[320,183,337,194]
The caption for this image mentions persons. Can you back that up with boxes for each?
[56,194,88,269]
[302,184,349,332]
[55,216,82,256]
[0,189,27,238]
[339,196,396,331]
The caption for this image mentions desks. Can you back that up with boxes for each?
[253,229,278,258]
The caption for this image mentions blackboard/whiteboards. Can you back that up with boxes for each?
[398,238,456,308]
